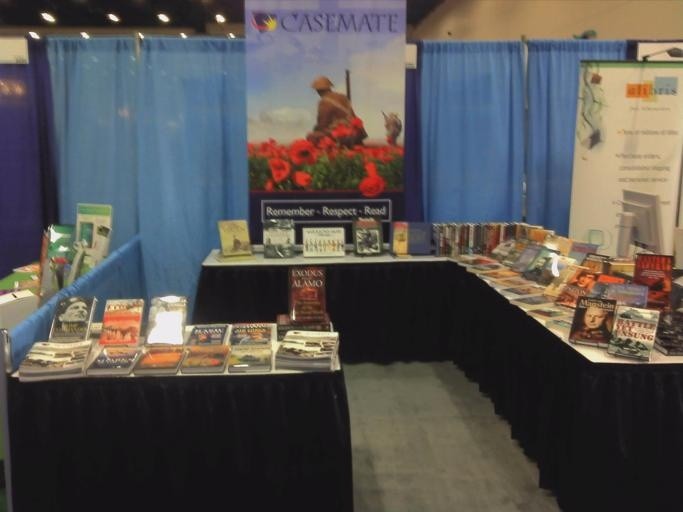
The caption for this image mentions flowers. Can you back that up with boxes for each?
[247,115,404,198]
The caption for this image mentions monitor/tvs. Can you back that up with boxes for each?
[611,190,665,263]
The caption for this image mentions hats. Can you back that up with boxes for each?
[312,75,333,89]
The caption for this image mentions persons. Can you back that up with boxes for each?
[304,76,367,150]
[384,112,402,147]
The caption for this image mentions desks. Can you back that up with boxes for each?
[7,246,683,512]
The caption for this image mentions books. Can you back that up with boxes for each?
[14,223,340,381]
[74,203,113,258]
[432,222,682,361]
[217,217,432,259]
